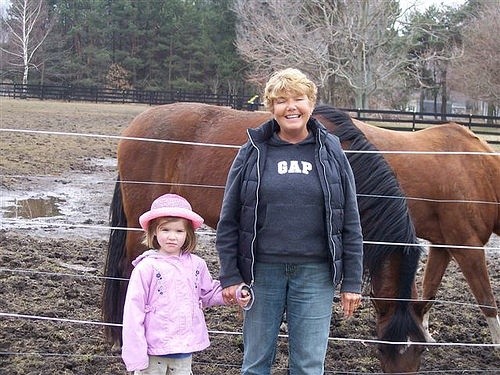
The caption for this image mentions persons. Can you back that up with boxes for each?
[122,194,254,375]
[215,67,364,375]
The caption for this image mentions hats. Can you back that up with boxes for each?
[139,193,204,232]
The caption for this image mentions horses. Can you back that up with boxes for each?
[97,102,434,375]
[350,118,500,356]
